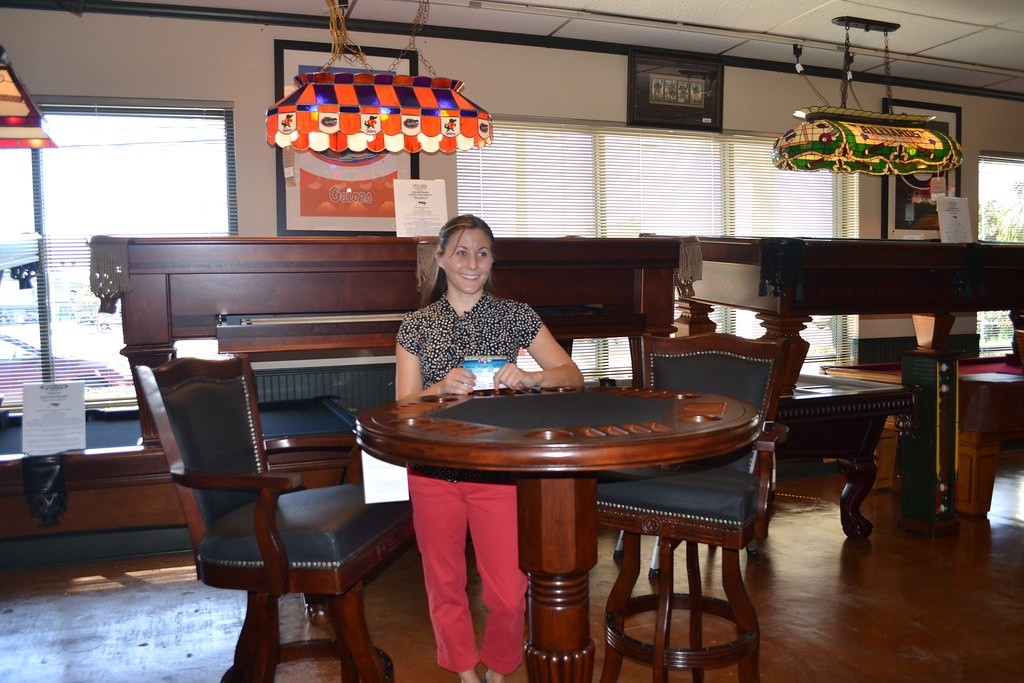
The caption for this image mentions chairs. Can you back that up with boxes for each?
[135,354,418,682]
[594,331,792,682]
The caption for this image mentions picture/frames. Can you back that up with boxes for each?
[272,37,420,235]
[771,16,963,176]
[625,45,726,133]
[880,97,964,242]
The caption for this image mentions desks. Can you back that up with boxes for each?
[1,230,1023,542]
[354,383,763,682]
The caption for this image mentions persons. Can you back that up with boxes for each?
[395,213,585,683]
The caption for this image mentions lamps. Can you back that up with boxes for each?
[264,0,493,155]
[1,45,60,150]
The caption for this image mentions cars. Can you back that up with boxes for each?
[1,330,132,408]
